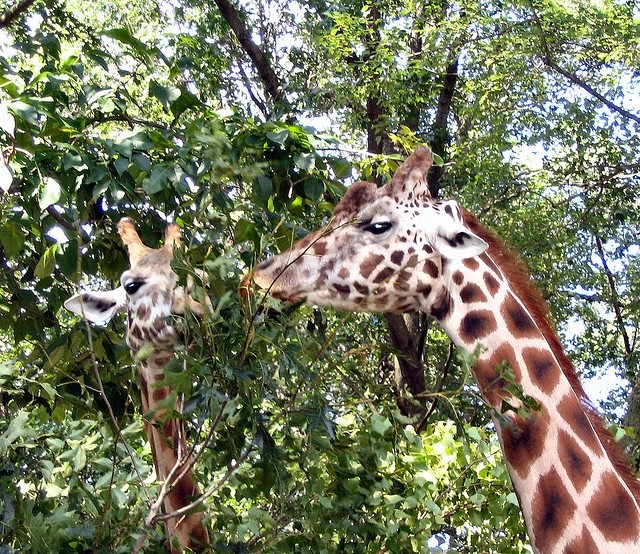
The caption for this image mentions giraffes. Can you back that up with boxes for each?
[237,144,639,552]
[63,216,215,553]
